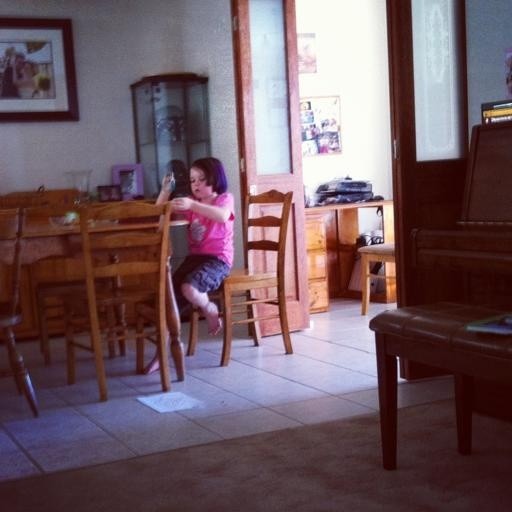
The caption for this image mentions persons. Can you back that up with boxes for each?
[302,113,339,154]
[141,157,236,376]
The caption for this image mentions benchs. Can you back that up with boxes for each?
[371,301,510,470]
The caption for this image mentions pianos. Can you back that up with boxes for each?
[410,101,512,457]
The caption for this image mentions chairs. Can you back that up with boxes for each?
[62,201,174,404]
[186,189,295,366]
[1,190,82,206]
[27,235,129,365]
[2,208,40,418]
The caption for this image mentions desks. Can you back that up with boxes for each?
[1,212,201,416]
[301,199,394,313]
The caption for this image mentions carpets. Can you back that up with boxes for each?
[2,398,511,511]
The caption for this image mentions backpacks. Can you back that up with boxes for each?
[315,179,373,206]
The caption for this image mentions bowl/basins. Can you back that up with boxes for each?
[47,216,74,230]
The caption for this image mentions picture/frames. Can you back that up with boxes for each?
[1,18,79,122]
[94,163,141,201]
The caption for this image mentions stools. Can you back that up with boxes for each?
[357,243,396,313]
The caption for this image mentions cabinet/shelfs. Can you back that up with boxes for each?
[131,74,212,201]
